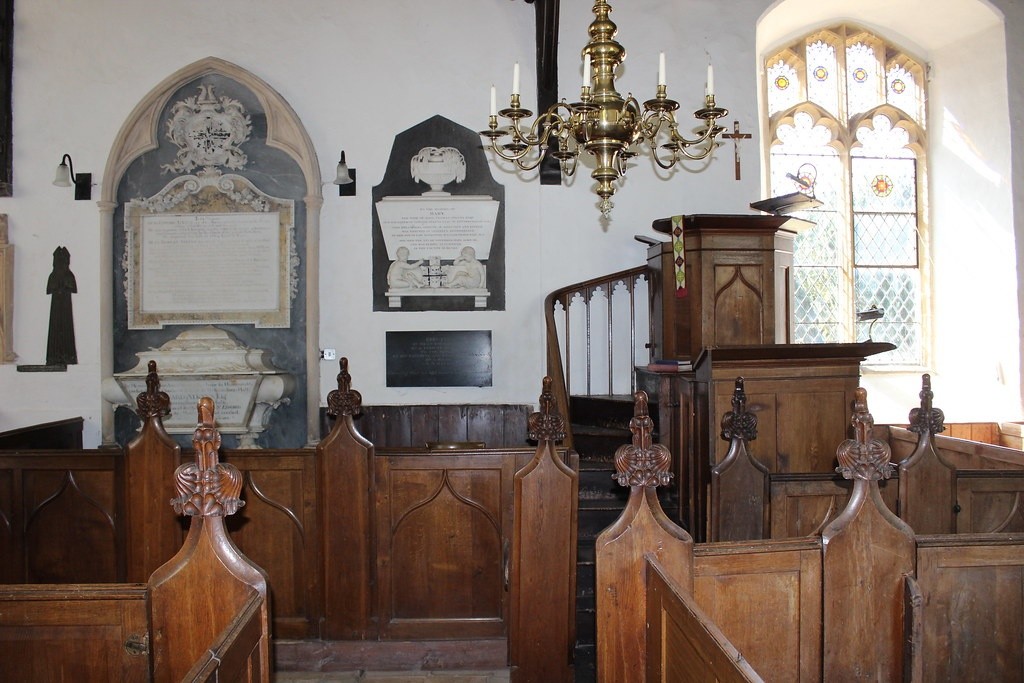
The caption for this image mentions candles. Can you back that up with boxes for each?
[704,82,708,98]
[707,63,714,94]
[659,50,665,84]
[490,83,496,114]
[512,60,520,94]
[583,51,591,86]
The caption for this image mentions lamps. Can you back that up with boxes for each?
[52,154,91,200]
[333,151,356,196]
